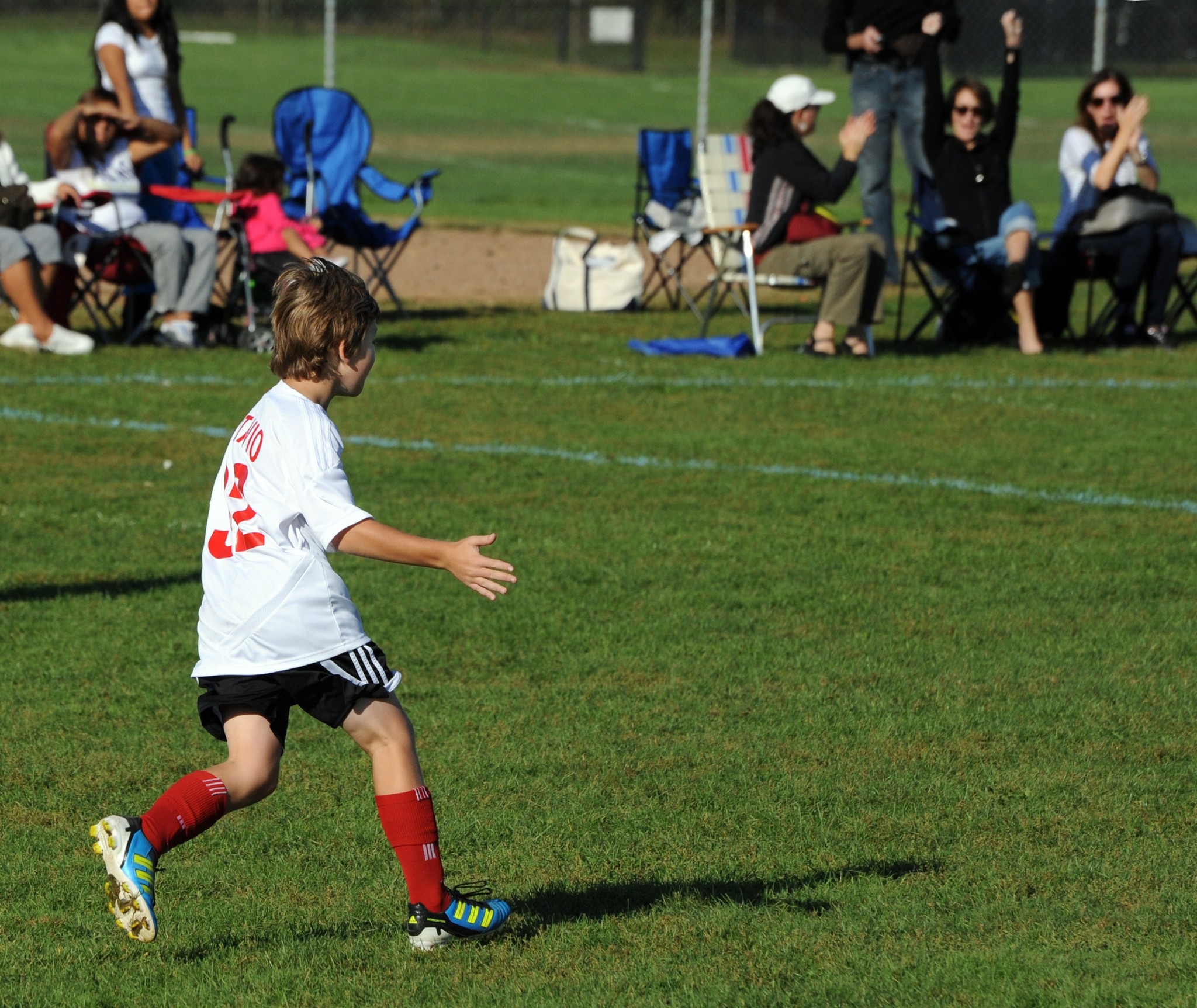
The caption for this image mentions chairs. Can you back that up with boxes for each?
[263,84,444,320]
[890,183,992,351]
[43,104,242,353]
[1054,209,1196,350]
[693,128,880,369]
[627,126,726,311]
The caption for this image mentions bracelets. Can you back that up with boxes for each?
[182,148,196,157]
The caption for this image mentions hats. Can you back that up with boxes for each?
[765,72,838,118]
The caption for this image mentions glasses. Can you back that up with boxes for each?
[950,105,988,116]
[1087,94,1124,109]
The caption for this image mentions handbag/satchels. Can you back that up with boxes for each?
[785,204,838,242]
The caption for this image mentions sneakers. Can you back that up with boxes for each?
[1,322,39,353]
[89,811,160,944]
[36,323,97,360]
[161,318,197,350]
[405,893,510,953]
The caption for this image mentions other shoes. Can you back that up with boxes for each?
[1143,325,1174,347]
[1115,323,1140,348]
[802,335,841,359]
[840,341,871,359]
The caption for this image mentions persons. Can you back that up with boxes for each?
[920,5,1052,355]
[1049,64,1176,348]
[45,88,218,348]
[738,75,879,363]
[0,131,96,357]
[207,152,349,342]
[81,256,517,960]
[822,0,960,291]
[91,0,207,232]
